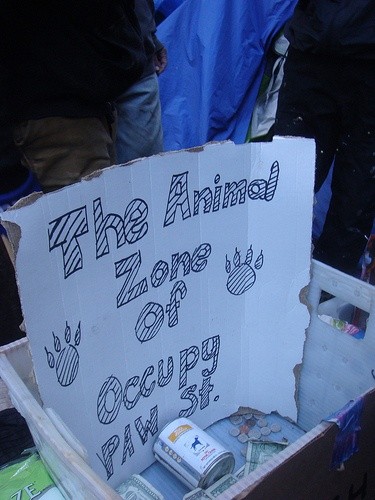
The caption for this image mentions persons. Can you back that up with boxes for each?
[283,0,375,305]
[0,0,144,346]
[113,0,170,164]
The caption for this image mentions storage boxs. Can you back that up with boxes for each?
[0,251,375,498]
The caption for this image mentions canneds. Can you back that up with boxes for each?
[152,417,235,490]
